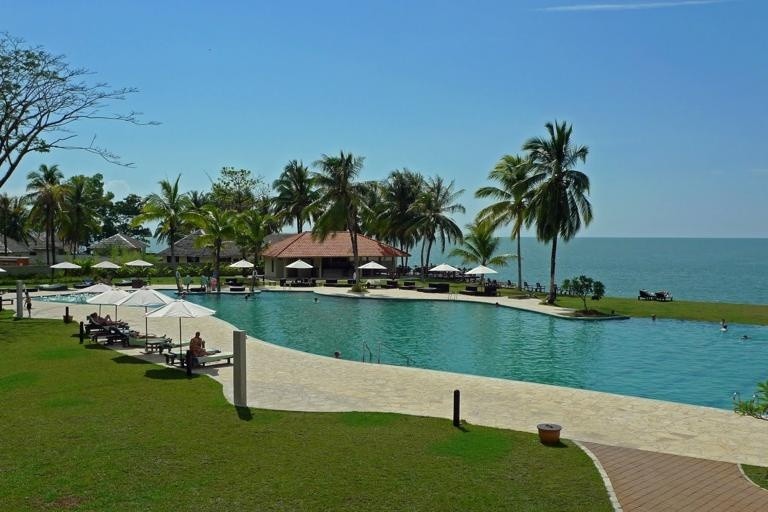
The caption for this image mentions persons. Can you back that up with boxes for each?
[92,311,131,329]
[185,331,220,358]
[716,318,750,341]
[20,291,33,316]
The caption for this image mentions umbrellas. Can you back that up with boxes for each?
[49,257,159,283]
[144,296,219,368]
[70,284,112,325]
[225,257,499,296]
[85,288,128,350]
[115,286,172,354]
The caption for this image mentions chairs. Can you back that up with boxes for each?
[637,289,672,302]
[279,277,498,297]
[82,313,172,350]
[164,347,234,368]
[38,280,147,291]
[488,278,575,297]
